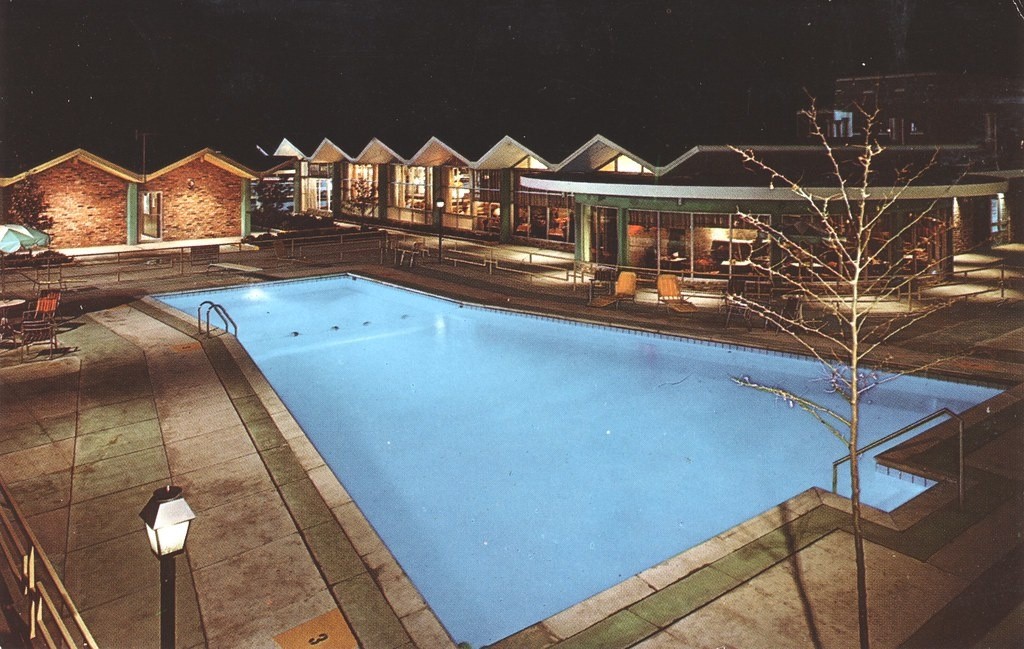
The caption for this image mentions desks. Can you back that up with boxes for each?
[0,298,26,347]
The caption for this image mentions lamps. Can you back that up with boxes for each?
[186,178,196,189]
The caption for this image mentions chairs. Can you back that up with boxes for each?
[16,271,89,364]
[653,273,701,318]
[716,276,800,328]
[206,262,263,277]
[589,271,639,310]
